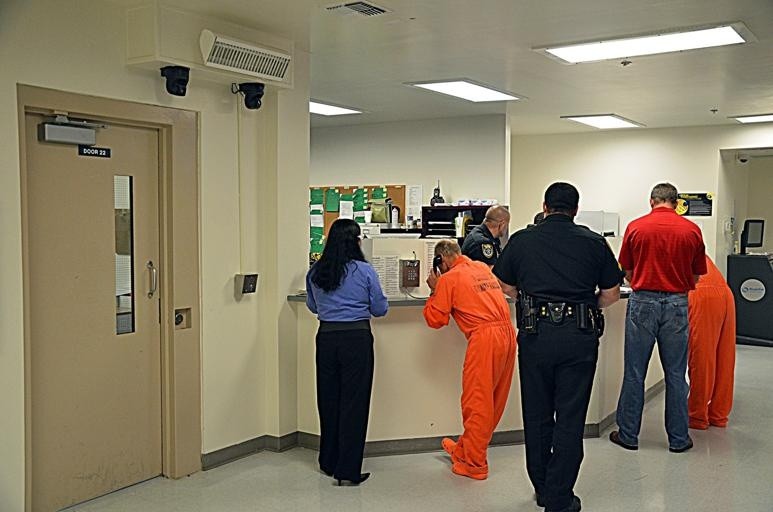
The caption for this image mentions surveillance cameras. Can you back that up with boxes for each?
[738,155,750,164]
[240,83,264,110]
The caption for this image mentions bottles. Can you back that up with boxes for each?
[392,207,421,229]
[734,240,739,255]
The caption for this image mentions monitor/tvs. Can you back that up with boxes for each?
[741,220,764,254]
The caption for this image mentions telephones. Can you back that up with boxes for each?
[433,254,442,274]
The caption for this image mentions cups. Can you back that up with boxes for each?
[455,216,464,238]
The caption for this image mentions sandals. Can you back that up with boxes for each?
[609,431,638,450]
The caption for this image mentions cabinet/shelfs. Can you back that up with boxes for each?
[422,205,509,247]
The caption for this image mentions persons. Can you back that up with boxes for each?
[306,219,389,486]
[423,239,516,480]
[461,205,510,269]
[687,255,736,430]
[610,183,707,453]
[492,182,623,512]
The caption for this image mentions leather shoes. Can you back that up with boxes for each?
[537,496,581,512]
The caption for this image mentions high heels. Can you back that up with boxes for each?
[326,473,370,486]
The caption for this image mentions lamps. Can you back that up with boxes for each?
[242,82,264,109]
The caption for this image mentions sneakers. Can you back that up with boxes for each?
[441,438,488,480]
[669,420,726,453]
